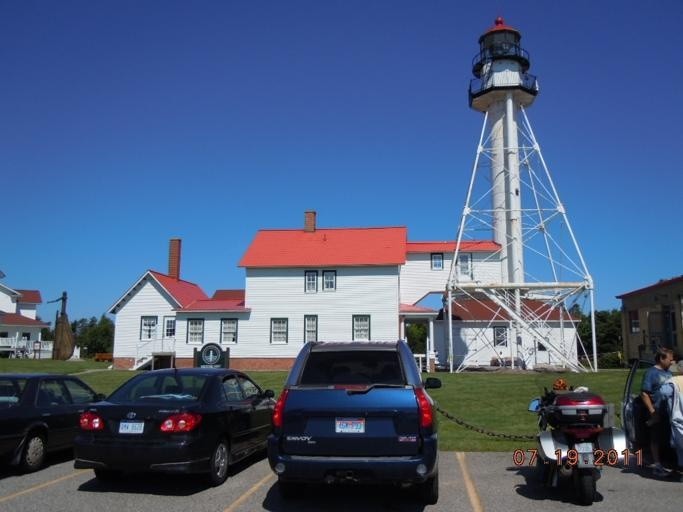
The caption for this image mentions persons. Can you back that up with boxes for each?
[639,347,674,476]
[652,360,683,474]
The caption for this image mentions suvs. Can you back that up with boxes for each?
[266,340,441,503]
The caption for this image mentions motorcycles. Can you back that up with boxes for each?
[527,386,609,506]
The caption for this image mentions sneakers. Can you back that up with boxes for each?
[652,462,672,477]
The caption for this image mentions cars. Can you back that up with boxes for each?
[71,366,277,485]
[619,359,682,472]
[1,373,105,478]
